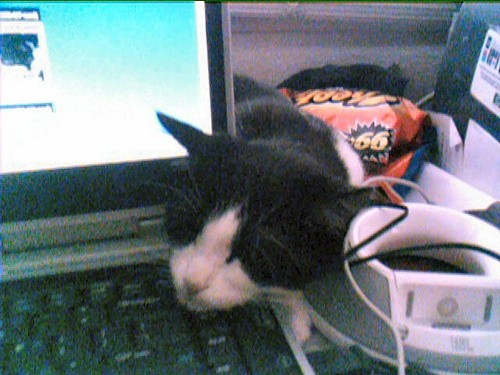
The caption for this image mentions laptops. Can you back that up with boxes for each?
[0,0,316,375]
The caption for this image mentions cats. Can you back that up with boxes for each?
[157,61,438,343]
